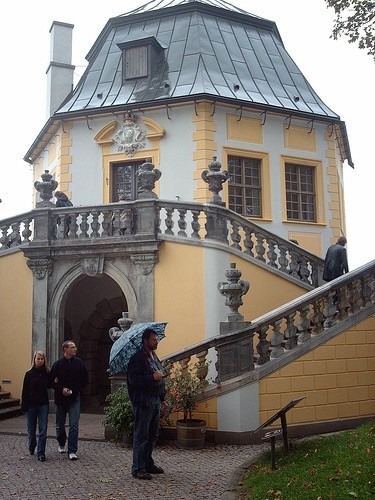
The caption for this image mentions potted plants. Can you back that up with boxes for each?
[103,385,135,447]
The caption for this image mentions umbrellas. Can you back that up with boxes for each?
[109,322,167,379]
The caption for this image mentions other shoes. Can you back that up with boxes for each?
[37,454,44,461]
[57,445,65,452]
[28,446,34,455]
[145,464,163,473]
[132,469,151,479]
[69,453,76,460]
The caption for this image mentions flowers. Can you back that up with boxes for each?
[159,363,221,426]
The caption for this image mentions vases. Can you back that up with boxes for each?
[177,418,205,449]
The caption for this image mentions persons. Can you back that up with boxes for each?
[126,329,167,480]
[20,351,59,462]
[321,236,349,318]
[49,340,89,460]
